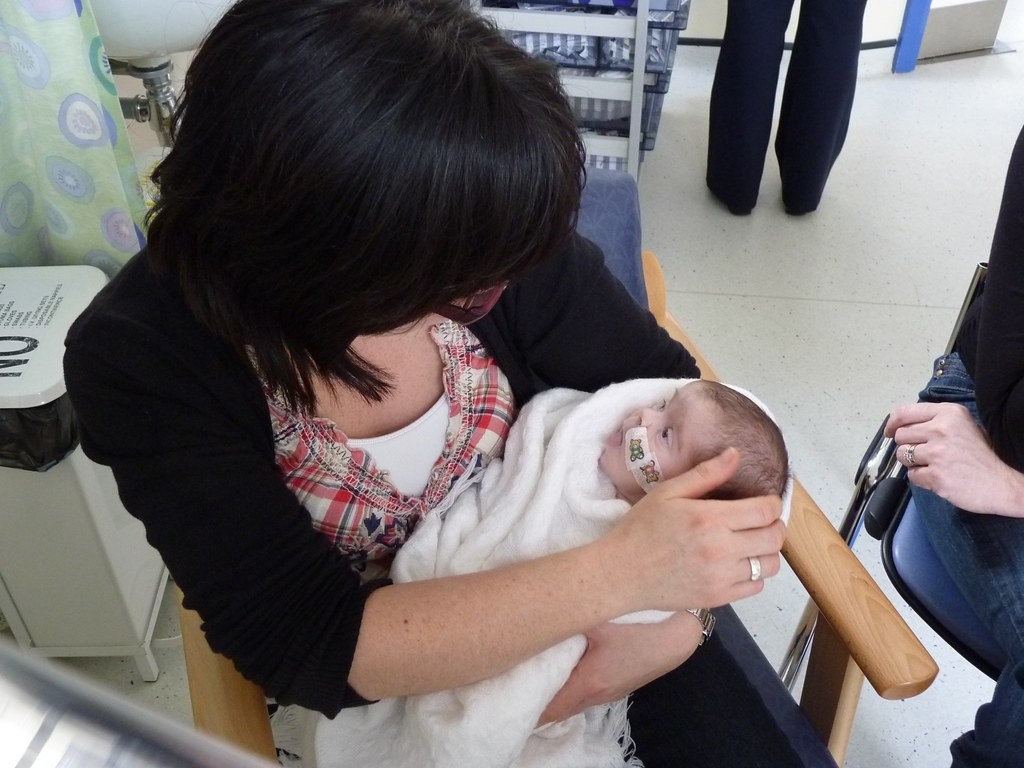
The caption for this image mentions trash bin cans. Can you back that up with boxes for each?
[0,260,172,684]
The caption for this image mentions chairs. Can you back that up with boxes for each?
[777,263,1006,768]
[178,168,939,768]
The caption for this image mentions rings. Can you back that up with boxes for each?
[748,555,762,581]
[904,444,917,463]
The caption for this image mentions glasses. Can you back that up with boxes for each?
[433,280,511,325]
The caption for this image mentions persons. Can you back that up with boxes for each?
[883,125,1024,768]
[707,0,868,216]
[64,1,839,767]
[272,378,794,767]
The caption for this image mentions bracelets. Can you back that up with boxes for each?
[687,607,717,646]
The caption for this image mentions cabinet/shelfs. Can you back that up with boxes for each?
[471,0,692,186]
